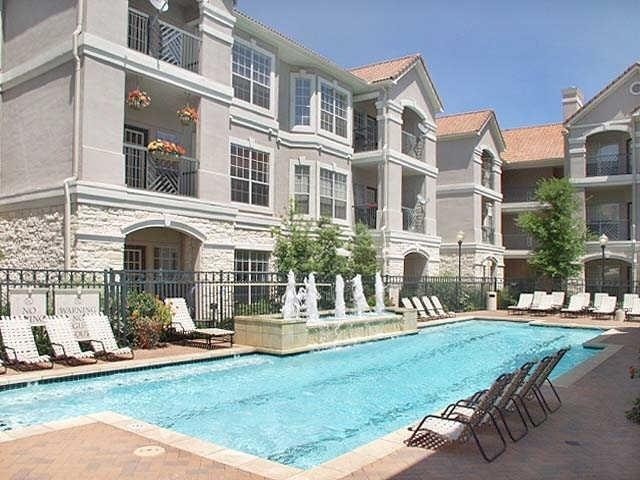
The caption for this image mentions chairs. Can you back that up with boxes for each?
[0,310,136,375]
[163,297,237,350]
[400,295,457,322]
[506,291,640,324]
[404,344,573,462]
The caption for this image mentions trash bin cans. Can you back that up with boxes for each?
[404,309,417,329]
[486,292,497,310]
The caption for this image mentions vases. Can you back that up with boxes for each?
[180,120,191,126]
[133,105,142,111]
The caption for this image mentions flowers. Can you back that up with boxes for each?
[175,106,199,124]
[147,139,186,160]
[125,90,152,111]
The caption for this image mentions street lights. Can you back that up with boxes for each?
[597,231,608,293]
[455,228,465,285]
